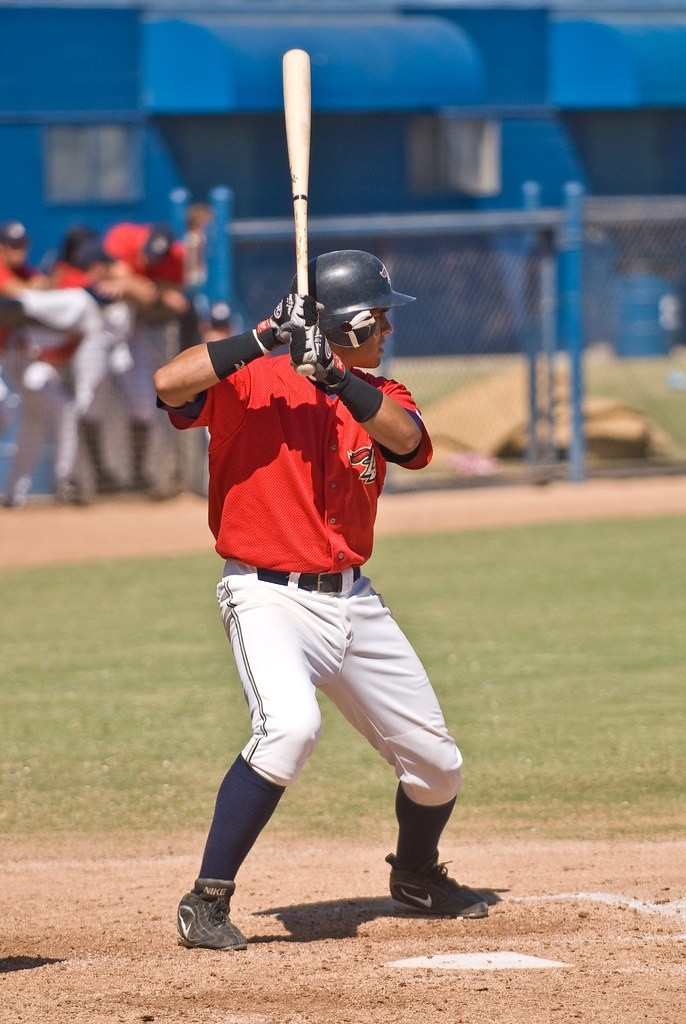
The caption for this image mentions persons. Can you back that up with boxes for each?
[0,202,210,509]
[154,250,491,950]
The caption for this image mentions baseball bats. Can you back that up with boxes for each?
[279,45,321,378]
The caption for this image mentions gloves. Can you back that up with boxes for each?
[256,292,324,352]
[276,322,348,395]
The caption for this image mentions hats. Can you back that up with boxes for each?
[4,226,28,246]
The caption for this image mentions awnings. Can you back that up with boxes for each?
[141,11,488,116]
[545,11,686,109]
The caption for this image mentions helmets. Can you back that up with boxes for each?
[291,250,417,348]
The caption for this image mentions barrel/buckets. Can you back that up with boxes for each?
[610,275,676,356]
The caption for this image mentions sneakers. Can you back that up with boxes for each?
[176,884,253,953]
[386,849,488,919]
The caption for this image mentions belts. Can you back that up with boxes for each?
[257,567,362,592]
